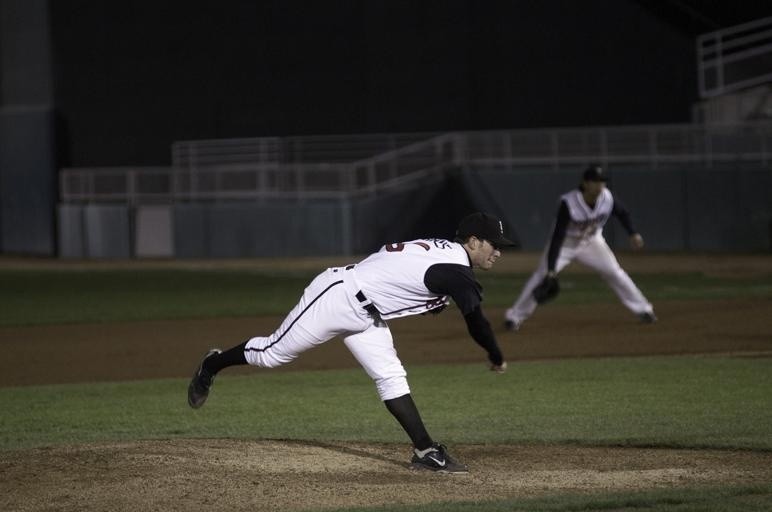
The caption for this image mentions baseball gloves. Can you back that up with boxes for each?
[532,276,560,304]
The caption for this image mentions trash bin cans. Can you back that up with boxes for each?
[132,207,173,257]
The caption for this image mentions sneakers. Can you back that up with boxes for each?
[410,441,471,474]
[186,347,225,409]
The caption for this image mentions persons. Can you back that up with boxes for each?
[501,162,660,333]
[187,210,515,476]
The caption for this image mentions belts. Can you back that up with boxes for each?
[345,262,382,321]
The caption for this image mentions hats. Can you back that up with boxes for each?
[457,208,521,248]
[582,164,610,183]
